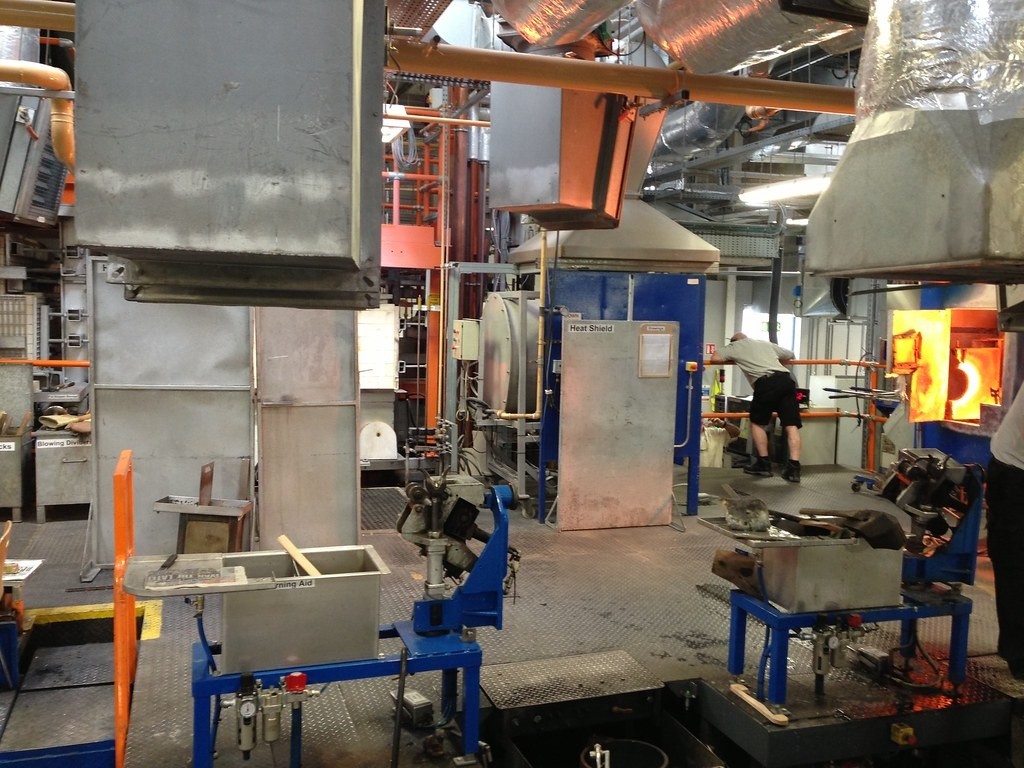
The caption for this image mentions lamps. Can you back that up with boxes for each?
[738,174,831,205]
[381,102,411,142]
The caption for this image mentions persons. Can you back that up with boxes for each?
[984,379,1024,681]
[710,333,804,483]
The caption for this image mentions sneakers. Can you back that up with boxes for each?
[782,460,801,482]
[744,458,772,477]
